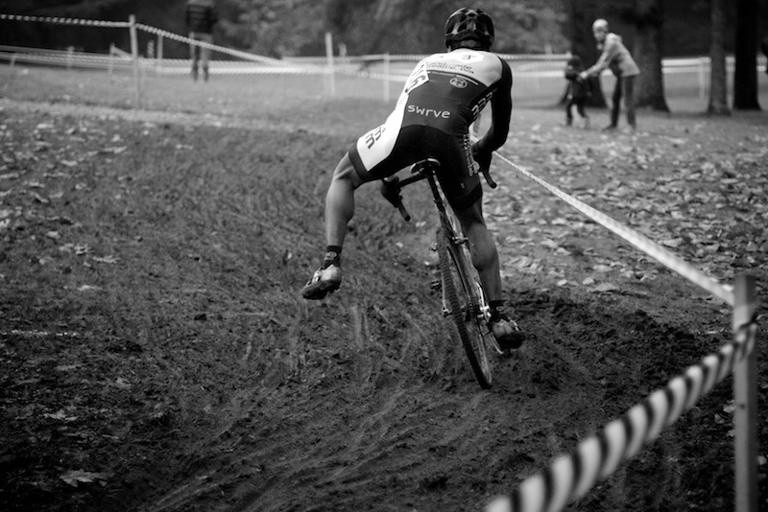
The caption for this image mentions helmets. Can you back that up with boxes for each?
[443,7,495,48]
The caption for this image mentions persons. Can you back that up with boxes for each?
[300,8,527,353]
[557,54,593,131]
[184,1,219,85]
[576,18,642,134]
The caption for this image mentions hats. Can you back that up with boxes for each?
[593,19,608,32]
[568,56,582,69]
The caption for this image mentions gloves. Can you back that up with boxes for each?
[382,175,403,208]
[471,140,491,167]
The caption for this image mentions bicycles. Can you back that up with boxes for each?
[379,148,526,390]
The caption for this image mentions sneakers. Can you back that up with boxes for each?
[301,260,342,299]
[565,115,636,135]
[492,316,525,349]
